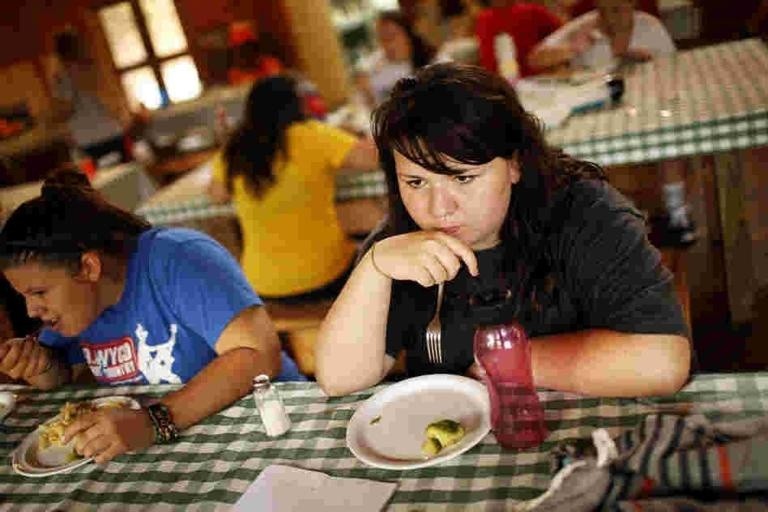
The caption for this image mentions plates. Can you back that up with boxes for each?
[1,391,18,420]
[11,394,142,480]
[344,372,500,471]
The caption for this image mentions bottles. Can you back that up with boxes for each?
[468,284,547,450]
[251,374,292,438]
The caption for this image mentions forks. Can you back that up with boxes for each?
[426,280,447,364]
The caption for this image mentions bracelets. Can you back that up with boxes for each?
[371,239,393,283]
[145,402,180,446]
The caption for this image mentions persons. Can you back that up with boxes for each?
[48,30,136,164]
[0,168,313,466]
[528,0,700,250]
[314,60,696,400]
[208,72,383,380]
[226,22,329,133]
[374,9,440,71]
[473,0,566,86]
